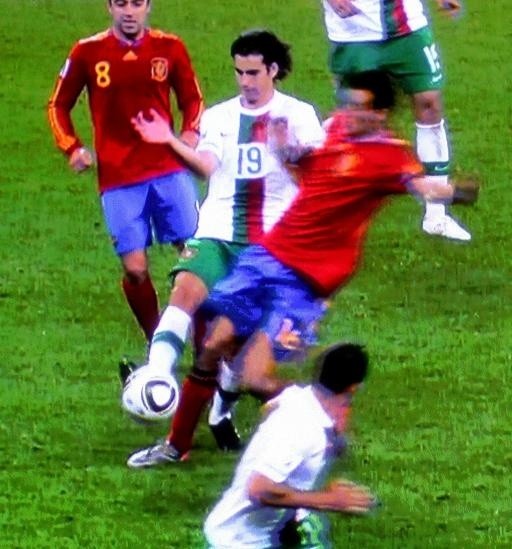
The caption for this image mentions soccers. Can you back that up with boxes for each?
[121,364,181,421]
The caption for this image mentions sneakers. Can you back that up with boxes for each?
[297,511,330,549]
[125,440,192,468]
[421,214,473,242]
[206,407,242,450]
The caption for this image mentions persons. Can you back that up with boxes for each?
[48,0,206,359]
[125,70,479,469]
[318,0,469,242]
[204,342,375,549]
[120,29,323,451]
